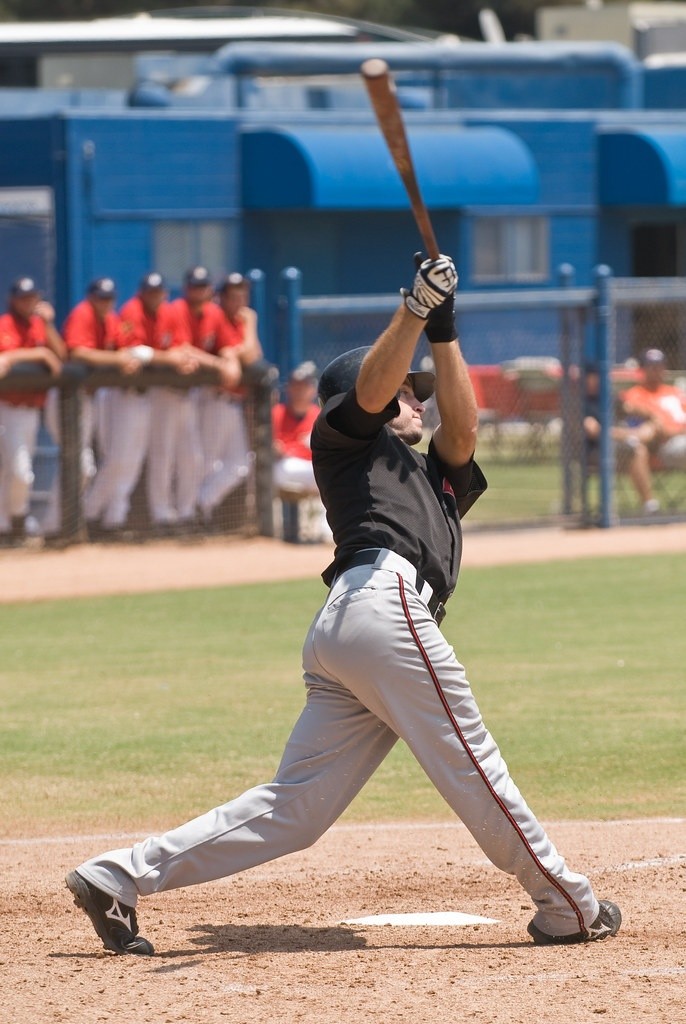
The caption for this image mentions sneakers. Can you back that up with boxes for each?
[527,900,622,945]
[65,870,155,956]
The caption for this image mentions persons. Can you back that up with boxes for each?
[0,73,686,550]
[65,253,623,955]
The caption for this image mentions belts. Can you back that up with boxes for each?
[334,549,446,628]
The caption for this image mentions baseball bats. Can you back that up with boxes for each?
[358,55,443,260]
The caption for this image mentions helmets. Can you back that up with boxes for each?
[317,346,435,409]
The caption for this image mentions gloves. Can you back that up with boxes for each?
[401,251,459,343]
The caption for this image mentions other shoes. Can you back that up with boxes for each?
[642,496,660,518]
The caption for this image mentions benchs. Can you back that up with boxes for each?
[580,371,686,525]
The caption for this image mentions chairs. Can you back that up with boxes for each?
[463,364,561,452]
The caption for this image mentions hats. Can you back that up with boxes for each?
[288,363,316,383]
[644,348,668,367]
[12,263,249,297]
